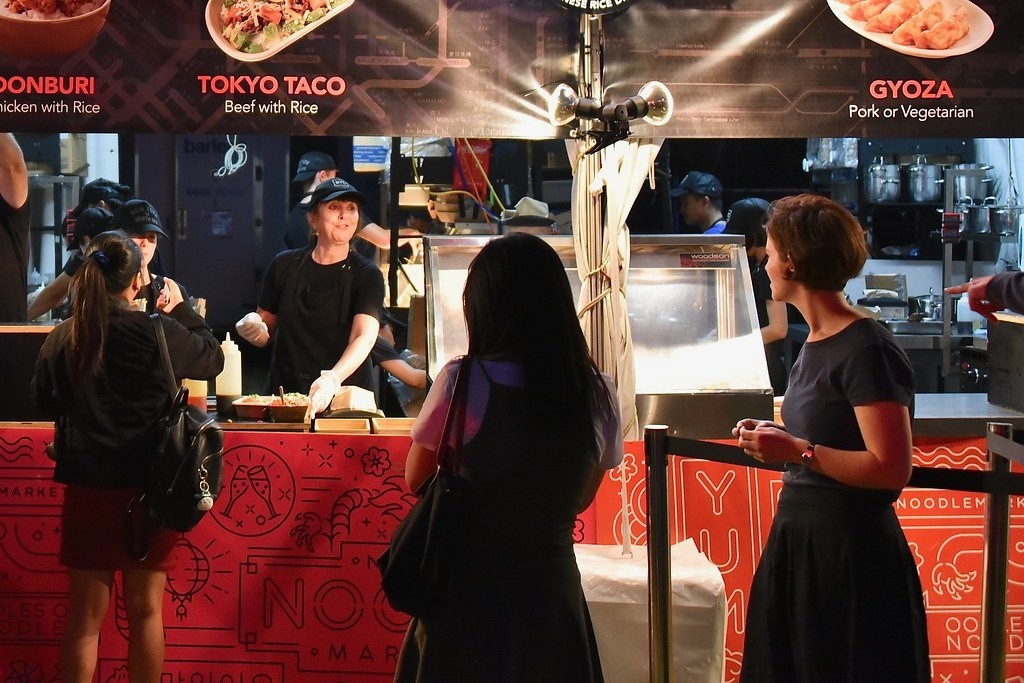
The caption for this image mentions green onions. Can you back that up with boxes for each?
[250,393,259,398]
[286,392,300,397]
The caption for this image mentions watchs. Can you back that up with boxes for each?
[801,443,815,468]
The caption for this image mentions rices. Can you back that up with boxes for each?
[0,0,106,20]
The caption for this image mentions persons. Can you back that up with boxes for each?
[343,308,427,410]
[732,194,932,683]
[235,178,385,424]
[391,230,623,683]
[37,232,225,683]
[285,152,416,250]
[722,198,787,393]
[27,178,133,321]
[945,271,1024,324]
[0,133,29,323]
[670,171,728,234]
[113,199,190,311]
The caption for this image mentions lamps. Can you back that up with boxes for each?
[549,80,674,155]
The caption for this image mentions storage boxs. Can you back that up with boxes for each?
[314,417,419,435]
[428,199,461,224]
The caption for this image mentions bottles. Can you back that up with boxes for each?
[28,266,42,294]
[215,331,242,421]
[352,135,389,173]
[184,378,208,415]
[35,281,47,322]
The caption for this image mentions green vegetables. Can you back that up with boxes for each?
[222,0,343,54]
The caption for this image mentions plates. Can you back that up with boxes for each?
[205,0,354,63]
[826,0,995,57]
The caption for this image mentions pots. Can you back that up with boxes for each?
[954,195,998,234]
[908,287,942,321]
[907,155,944,202]
[861,155,902,203]
[951,162,995,201]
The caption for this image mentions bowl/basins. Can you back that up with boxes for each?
[314,417,370,434]
[269,395,308,423]
[372,417,417,433]
[232,395,274,422]
[0,0,112,56]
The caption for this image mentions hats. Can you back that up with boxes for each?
[112,200,168,240]
[66,204,114,250]
[378,307,408,329]
[291,150,337,184]
[311,177,365,204]
[671,170,720,199]
[70,184,119,218]
[719,196,771,245]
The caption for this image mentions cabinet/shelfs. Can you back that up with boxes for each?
[26,176,85,320]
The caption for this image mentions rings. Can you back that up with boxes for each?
[970,278,974,283]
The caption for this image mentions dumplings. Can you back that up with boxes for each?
[836,0,970,50]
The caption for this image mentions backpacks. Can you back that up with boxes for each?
[125,311,223,561]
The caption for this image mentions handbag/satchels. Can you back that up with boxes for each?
[380,356,471,616]
